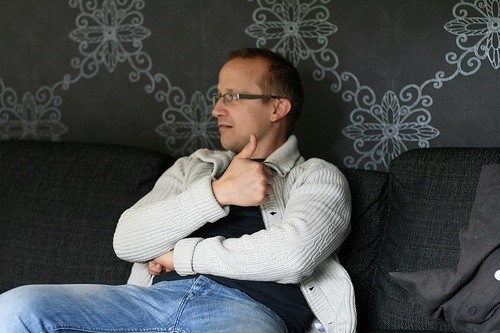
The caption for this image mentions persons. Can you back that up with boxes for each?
[0,47,356,333]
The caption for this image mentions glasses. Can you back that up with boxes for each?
[212,92,283,107]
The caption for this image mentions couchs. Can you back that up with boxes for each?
[0,138,500,333]
